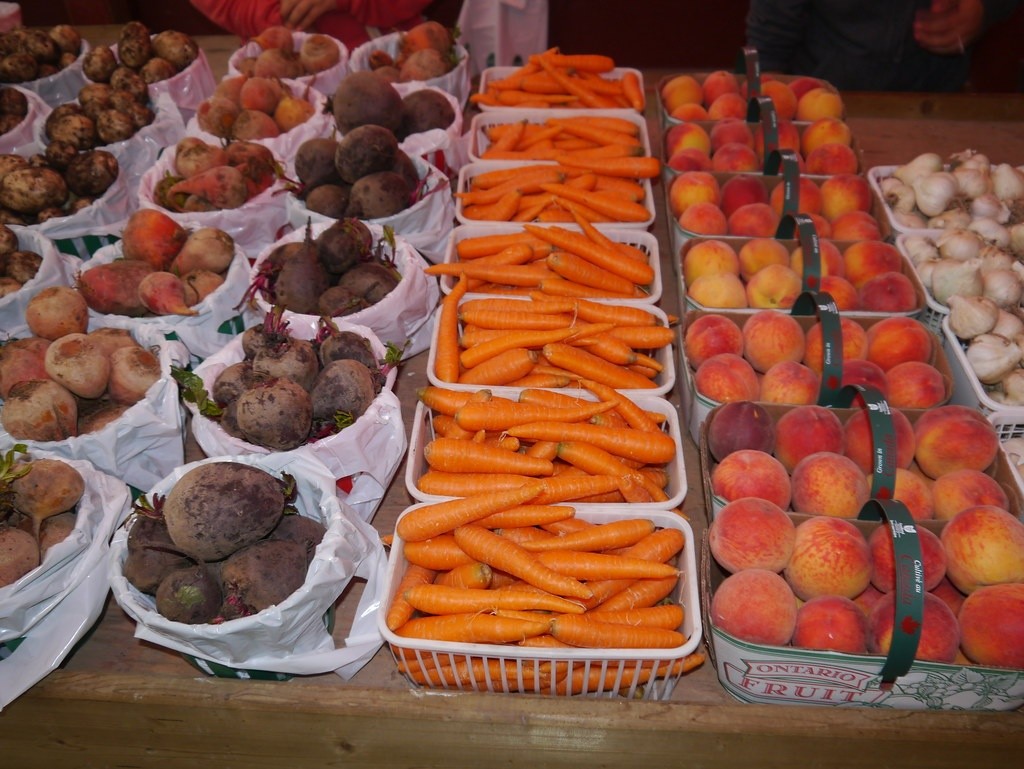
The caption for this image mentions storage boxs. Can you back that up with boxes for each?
[384,45,1024,710]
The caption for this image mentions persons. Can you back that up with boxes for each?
[190,0,437,40]
[746,0,1022,87]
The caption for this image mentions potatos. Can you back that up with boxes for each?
[0,20,458,632]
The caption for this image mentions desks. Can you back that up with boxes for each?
[0,25,1024,768]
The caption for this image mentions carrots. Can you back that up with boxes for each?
[382,46,708,690]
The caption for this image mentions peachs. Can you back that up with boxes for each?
[661,69,1023,668]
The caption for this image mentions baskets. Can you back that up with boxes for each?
[478,65,646,117]
[454,161,656,231]
[866,160,1024,234]
[891,227,1024,344]
[377,500,704,696]
[467,110,651,164]
[427,297,676,396]
[984,408,1024,525]
[934,311,1024,418]
[439,224,662,306]
[404,387,687,511]
